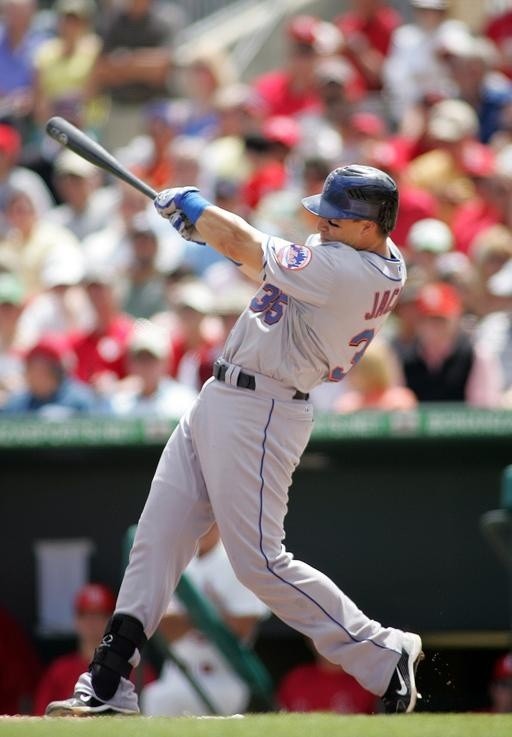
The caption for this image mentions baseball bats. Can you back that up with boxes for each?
[44,116,159,199]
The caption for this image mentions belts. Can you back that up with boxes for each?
[212,358,308,401]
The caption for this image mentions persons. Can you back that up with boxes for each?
[30,580,156,716]
[0,0,512,425]
[137,512,274,713]
[45,162,425,719]
[278,633,380,715]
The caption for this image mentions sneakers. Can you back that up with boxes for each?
[377,629,424,714]
[45,669,140,715]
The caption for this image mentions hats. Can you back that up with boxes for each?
[71,584,115,615]
[0,1,511,364]
